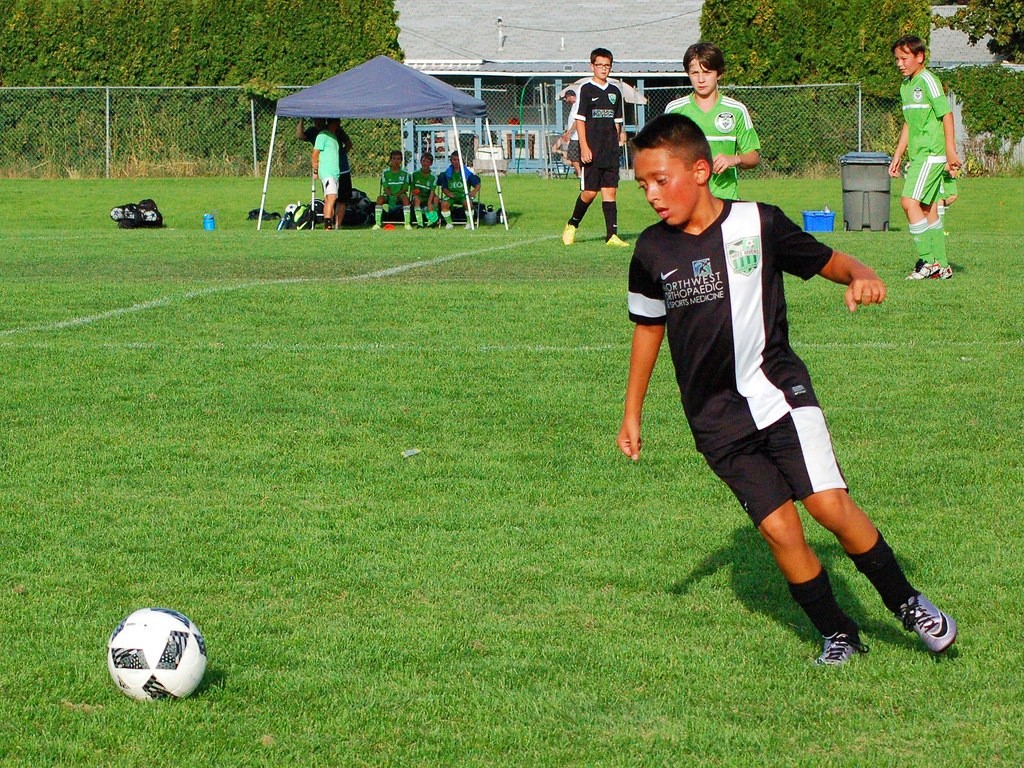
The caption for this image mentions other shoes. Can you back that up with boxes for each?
[373,225,381,230]
[464,224,470,229]
[446,223,454,230]
[404,225,412,230]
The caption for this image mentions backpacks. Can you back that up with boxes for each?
[248,193,324,231]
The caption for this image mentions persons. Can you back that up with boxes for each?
[559,89,582,179]
[312,117,341,231]
[617,112,957,667]
[888,35,961,279]
[440,150,481,229]
[551,129,572,165]
[409,153,439,229]
[372,150,413,230]
[664,42,760,201]
[562,48,631,246]
[296,116,353,230]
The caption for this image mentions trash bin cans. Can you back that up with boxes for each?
[839,151,893,232]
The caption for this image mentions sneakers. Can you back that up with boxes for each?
[905,258,940,281]
[894,591,957,652]
[815,620,869,665]
[564,219,578,244]
[606,233,629,246]
[929,266,953,280]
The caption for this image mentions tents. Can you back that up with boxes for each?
[255,55,510,231]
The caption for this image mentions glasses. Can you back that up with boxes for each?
[594,63,612,69]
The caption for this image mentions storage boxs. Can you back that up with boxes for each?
[801,211,837,233]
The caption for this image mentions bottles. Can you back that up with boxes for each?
[204,214,214,230]
[484,204,507,224]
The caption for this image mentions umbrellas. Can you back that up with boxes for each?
[554,76,648,106]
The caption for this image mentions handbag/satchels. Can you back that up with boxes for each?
[109,198,164,230]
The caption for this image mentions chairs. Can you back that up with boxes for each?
[546,132,569,178]
[378,173,482,229]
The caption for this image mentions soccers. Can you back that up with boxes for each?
[106,606,210,701]
[285,203,297,214]
[110,199,160,228]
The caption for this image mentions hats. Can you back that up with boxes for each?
[560,90,575,100]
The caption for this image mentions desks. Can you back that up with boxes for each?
[506,134,535,160]
